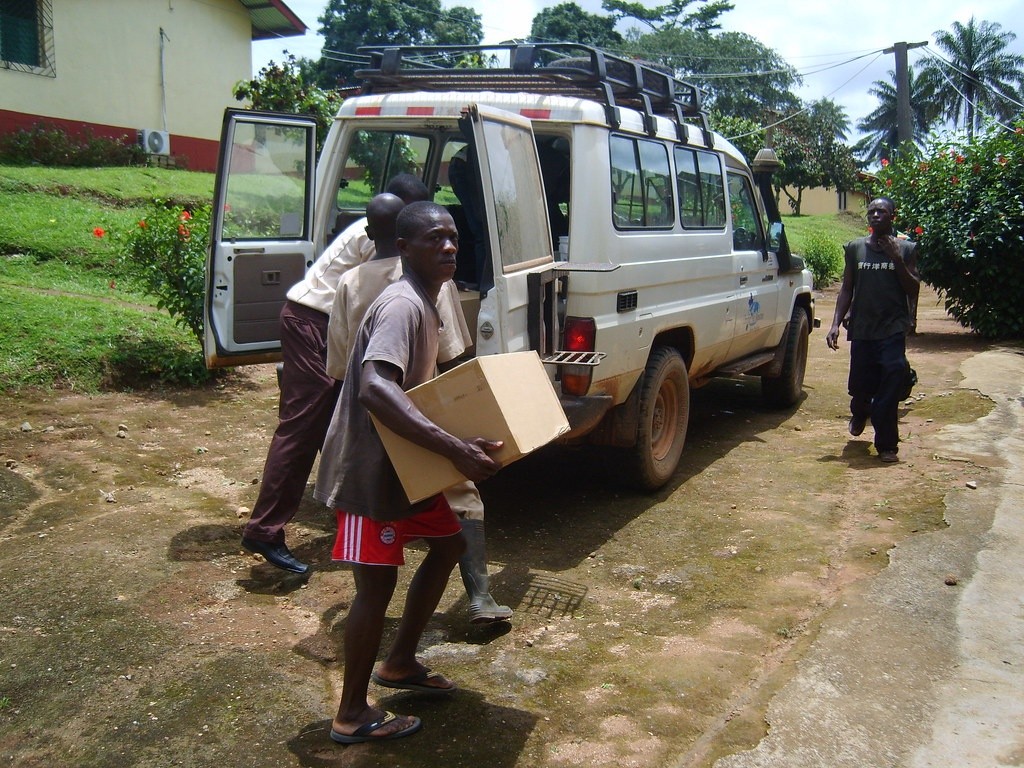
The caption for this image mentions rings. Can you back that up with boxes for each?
[877,241,880,246]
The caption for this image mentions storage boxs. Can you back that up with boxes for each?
[368,351,571,506]
[458,289,481,356]
[559,236,569,261]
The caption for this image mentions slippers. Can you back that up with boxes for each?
[373,667,457,693]
[330,711,422,742]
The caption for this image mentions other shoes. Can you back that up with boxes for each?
[849,416,865,436]
[879,450,898,461]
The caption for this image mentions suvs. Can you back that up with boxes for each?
[203,37,823,496]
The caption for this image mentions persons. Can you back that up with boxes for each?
[449,138,572,292]
[312,201,504,745]
[239,173,434,574]
[826,196,926,461]
[325,191,513,625]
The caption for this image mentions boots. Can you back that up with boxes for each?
[459,520,513,624]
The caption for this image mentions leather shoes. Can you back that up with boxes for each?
[241,537,308,573]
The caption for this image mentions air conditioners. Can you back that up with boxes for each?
[137,129,170,156]
